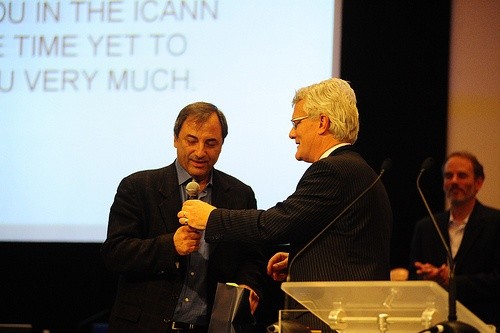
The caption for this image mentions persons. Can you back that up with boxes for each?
[177,78,392,333]
[406,151,500,333]
[102,101,268,333]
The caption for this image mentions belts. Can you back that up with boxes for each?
[170,320,207,332]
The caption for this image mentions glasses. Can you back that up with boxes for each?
[291,114,332,130]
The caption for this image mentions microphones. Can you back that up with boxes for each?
[284,157,391,319]
[186,181,200,201]
[416,155,480,333]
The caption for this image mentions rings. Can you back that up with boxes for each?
[183,211,186,217]
[185,218,188,225]
